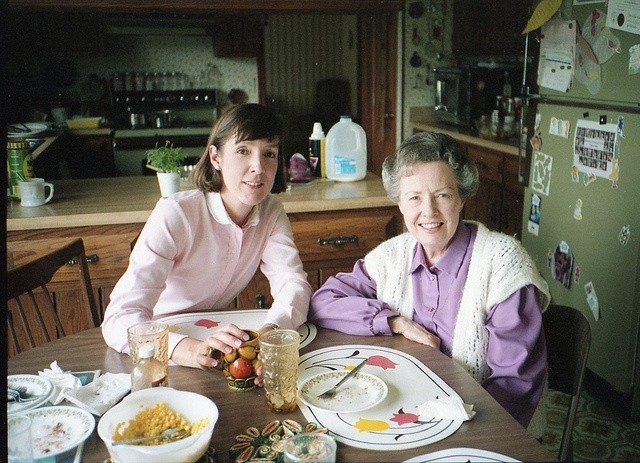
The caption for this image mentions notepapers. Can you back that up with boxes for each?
[521,0,562,34]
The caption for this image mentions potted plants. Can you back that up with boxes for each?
[146,140,186,196]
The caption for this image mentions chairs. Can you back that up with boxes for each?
[8,237,100,360]
[543,307,591,463]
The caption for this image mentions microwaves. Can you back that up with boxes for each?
[431,65,499,116]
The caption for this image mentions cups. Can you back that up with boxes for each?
[18,177,54,206]
[127,322,169,392]
[207,329,263,392]
[283,432,337,462]
[258,328,300,414]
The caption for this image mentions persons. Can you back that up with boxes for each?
[310,131,550,447]
[101,103,314,372]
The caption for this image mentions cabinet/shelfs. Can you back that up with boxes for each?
[236,216,394,311]
[7,223,145,361]
[463,144,523,241]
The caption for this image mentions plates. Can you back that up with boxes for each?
[7,122,47,137]
[8,374,56,413]
[8,406,96,459]
[297,370,388,413]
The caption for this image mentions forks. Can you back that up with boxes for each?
[111,426,192,446]
[317,359,368,400]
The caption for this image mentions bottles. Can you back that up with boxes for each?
[325,115,368,181]
[480,70,527,145]
[309,121,326,180]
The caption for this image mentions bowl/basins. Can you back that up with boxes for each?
[97,387,219,462]
